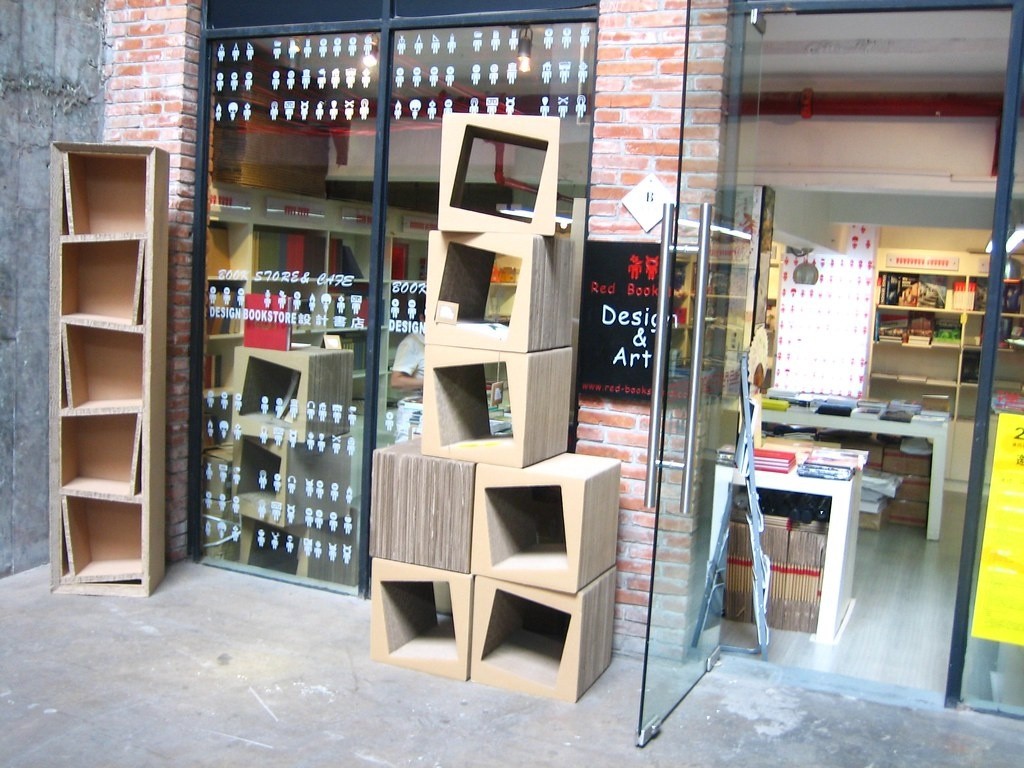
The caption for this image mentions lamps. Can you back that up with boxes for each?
[362,34,378,68]
[516,28,533,73]
[793,248,819,285]
[985,223,1024,255]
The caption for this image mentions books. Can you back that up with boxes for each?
[979,315,1024,350]
[753,448,797,474]
[878,312,960,349]
[877,271,976,311]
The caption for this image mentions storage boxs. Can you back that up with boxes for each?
[200,441,239,563]
[818,436,930,532]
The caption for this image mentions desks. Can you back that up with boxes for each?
[762,410,950,541]
[704,443,865,643]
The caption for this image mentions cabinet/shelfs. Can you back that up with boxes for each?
[49,142,170,598]
[203,214,429,450]
[864,266,1024,484]
